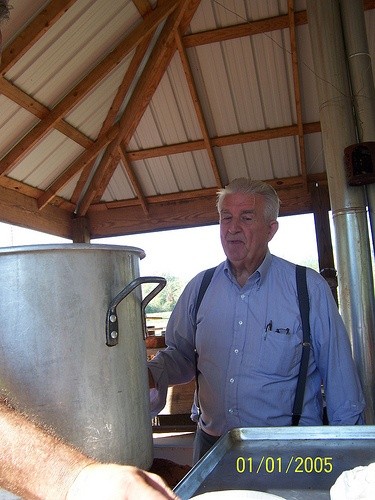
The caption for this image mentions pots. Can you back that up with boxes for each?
[1,243,168,470]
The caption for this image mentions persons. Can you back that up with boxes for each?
[1,360,180,500]
[144,175,367,478]
